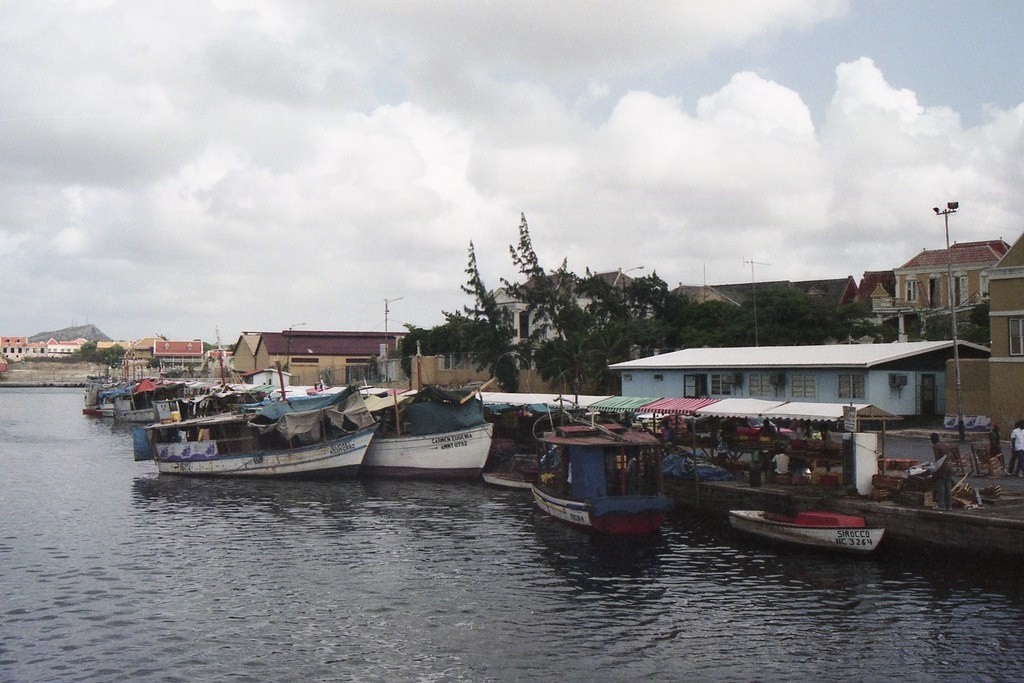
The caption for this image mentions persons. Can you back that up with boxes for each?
[988,423,1005,474]
[658,419,832,489]
[1008,419,1024,477]
[626,453,641,493]
[930,432,953,512]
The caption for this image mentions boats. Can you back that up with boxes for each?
[361,389,494,482]
[82,374,264,420]
[728,506,886,554]
[133,383,374,476]
[530,403,673,536]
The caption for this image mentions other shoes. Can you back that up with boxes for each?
[933,506,947,511]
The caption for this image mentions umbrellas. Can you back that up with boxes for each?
[132,379,156,408]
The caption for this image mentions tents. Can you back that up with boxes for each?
[589,395,902,420]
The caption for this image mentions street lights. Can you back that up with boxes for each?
[383,296,403,343]
[933,202,965,438]
[289,322,304,366]
[621,265,645,313]
